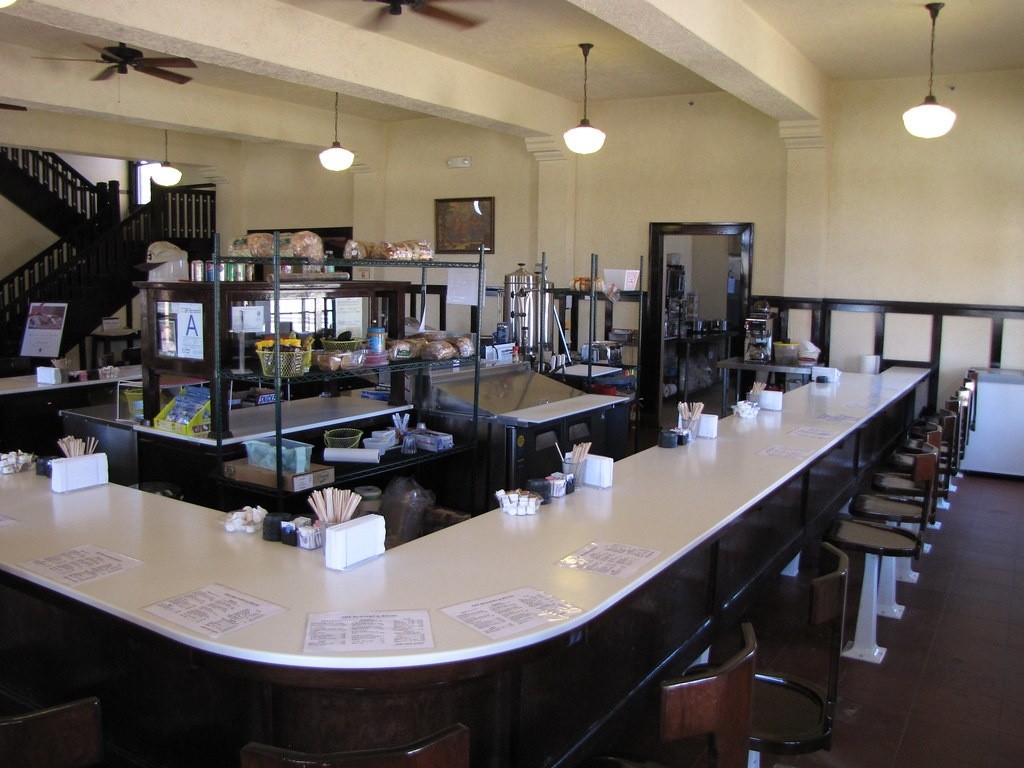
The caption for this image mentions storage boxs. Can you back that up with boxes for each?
[244,436,315,474]
[223,457,334,493]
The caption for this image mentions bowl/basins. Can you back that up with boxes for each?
[363,429,397,456]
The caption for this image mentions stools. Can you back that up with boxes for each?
[589,370,978,768]
[0,695,103,768]
[240,722,470,768]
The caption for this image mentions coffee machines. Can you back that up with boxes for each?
[743,318,773,364]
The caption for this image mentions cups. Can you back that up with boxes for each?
[319,522,336,556]
[562,458,587,491]
[746,393,760,407]
[681,419,698,439]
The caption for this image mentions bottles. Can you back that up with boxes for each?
[220,258,255,282]
[324,251,335,273]
[732,401,760,419]
[816,376,828,388]
[401,431,417,453]
[495,472,575,515]
[226,504,291,541]
[513,345,519,365]
[658,427,694,448]
[581,343,623,366]
[496,322,509,343]
[0,449,61,477]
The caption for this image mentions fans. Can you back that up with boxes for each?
[30,42,197,84]
[364,0,483,29]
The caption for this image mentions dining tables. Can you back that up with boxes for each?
[0,365,143,457]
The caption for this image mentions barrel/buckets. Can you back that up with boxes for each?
[124,387,143,418]
[366,328,386,353]
[773,339,821,366]
[323,429,362,449]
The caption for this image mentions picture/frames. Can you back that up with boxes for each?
[435,197,495,254]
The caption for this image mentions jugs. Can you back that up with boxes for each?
[745,343,768,360]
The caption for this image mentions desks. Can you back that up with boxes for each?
[716,356,825,418]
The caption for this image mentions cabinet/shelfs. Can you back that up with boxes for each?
[213,232,485,517]
[133,281,410,469]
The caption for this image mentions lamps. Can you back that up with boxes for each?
[903,3,957,139]
[319,92,354,171]
[151,129,182,187]
[563,44,607,153]
